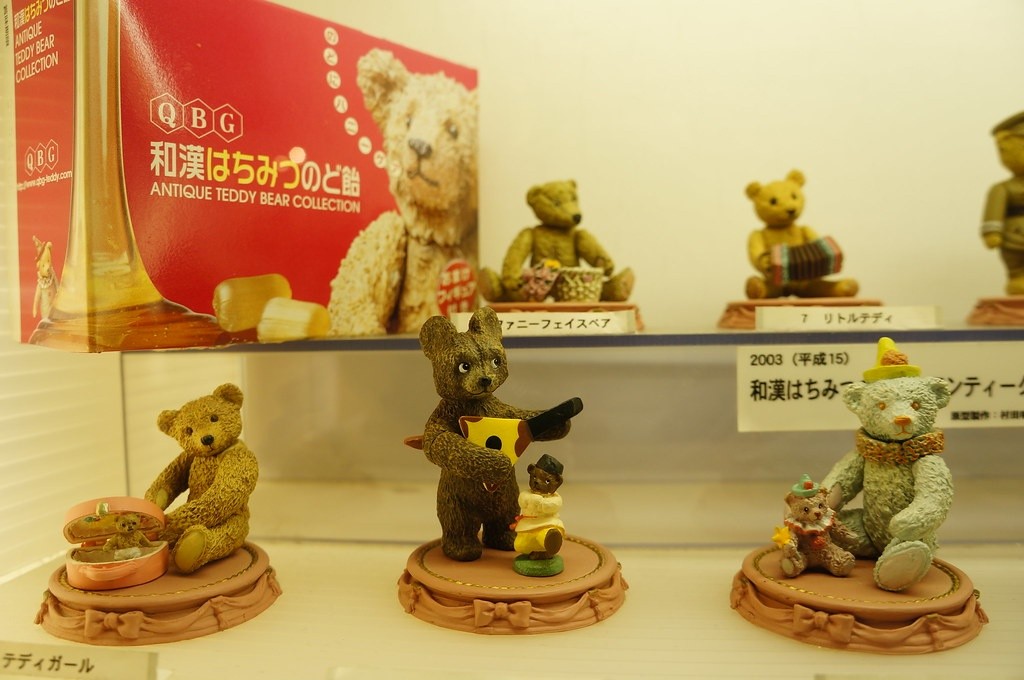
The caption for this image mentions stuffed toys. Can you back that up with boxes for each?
[103,513,154,560]
[479,179,634,303]
[144,383,259,572]
[745,170,859,298]
[779,473,860,578]
[821,348,954,591]
[510,453,565,560]
[980,112,1024,293]
[327,47,481,335]
[420,307,571,561]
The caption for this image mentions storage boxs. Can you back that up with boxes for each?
[0,0,479,354]
